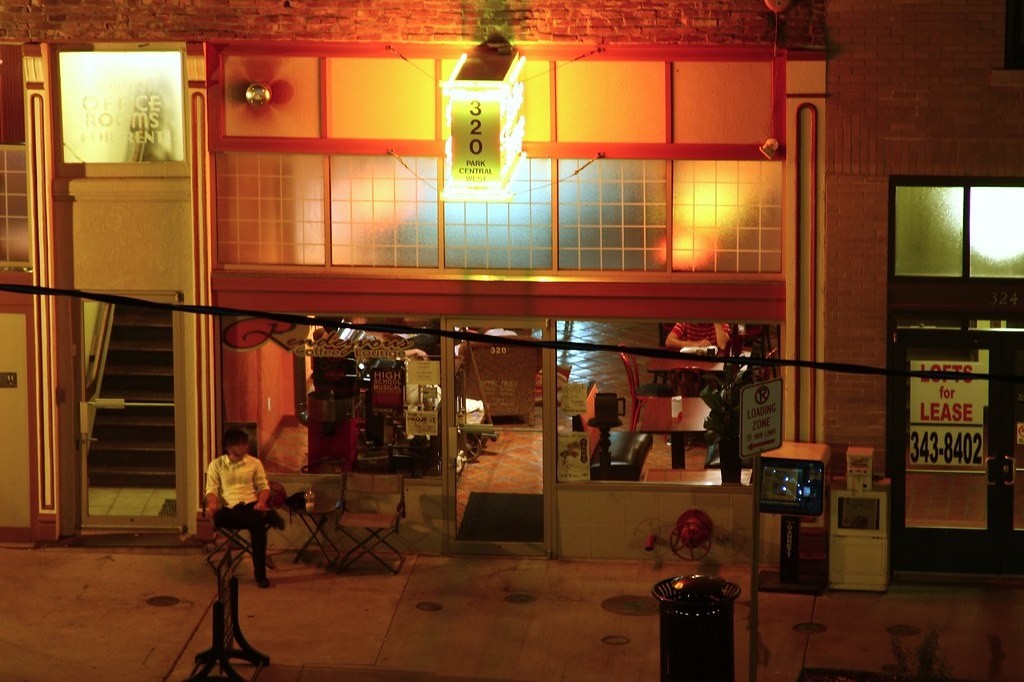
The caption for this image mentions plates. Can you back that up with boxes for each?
[680,345,718,355]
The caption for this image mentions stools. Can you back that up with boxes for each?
[203,515,278,576]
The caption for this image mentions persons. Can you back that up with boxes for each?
[205,428,285,589]
[345,317,429,361]
[481,327,518,336]
[665,322,732,397]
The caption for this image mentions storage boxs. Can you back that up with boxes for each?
[406,400,441,436]
[345,491,405,516]
[345,472,404,493]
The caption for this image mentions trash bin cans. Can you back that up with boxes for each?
[651,575,741,682]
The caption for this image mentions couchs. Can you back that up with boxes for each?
[455,336,543,425]
[582,381,653,484]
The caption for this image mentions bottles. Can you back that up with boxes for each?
[671,395,682,419]
[696,348,715,356]
[304,485,315,514]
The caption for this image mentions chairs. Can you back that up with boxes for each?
[619,342,673,433]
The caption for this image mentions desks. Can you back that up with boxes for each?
[646,348,739,373]
[639,397,709,468]
[293,498,340,567]
[647,467,751,487]
[330,510,406,575]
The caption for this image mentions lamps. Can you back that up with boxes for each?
[246,82,275,108]
[759,137,781,163]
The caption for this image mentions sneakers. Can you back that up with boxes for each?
[267,510,286,531]
[255,571,269,588]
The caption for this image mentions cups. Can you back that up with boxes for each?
[593,392,627,424]
